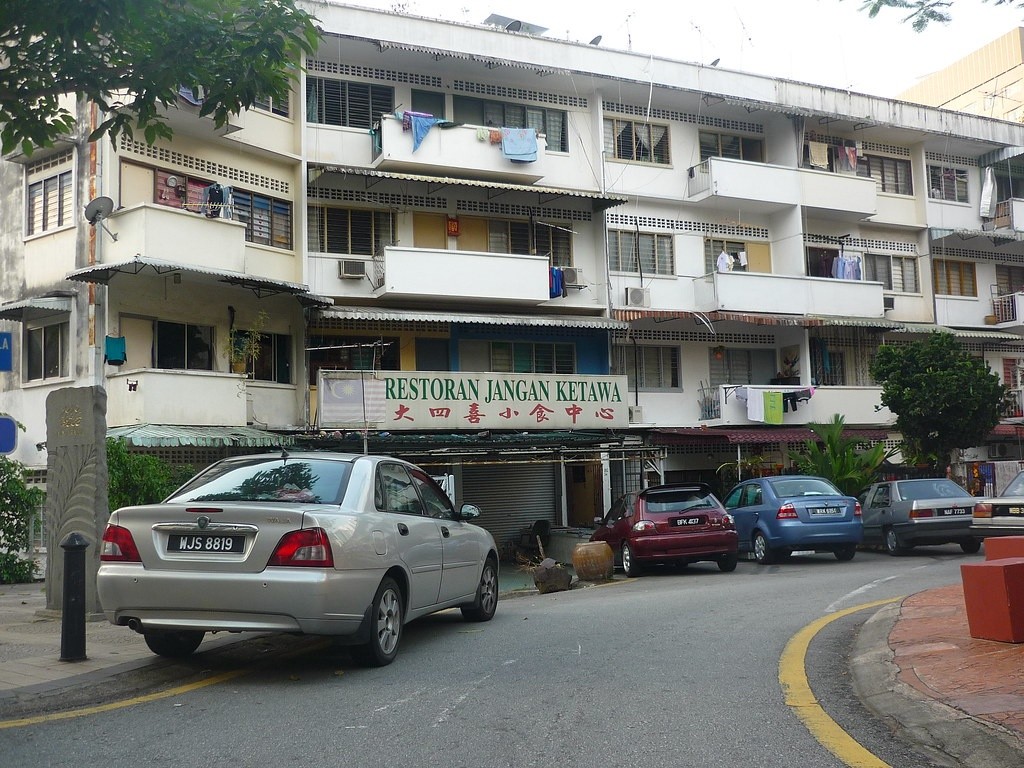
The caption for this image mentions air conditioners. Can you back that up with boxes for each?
[625,287,652,308]
[336,260,367,280]
[628,405,643,424]
[991,443,1010,457]
[560,266,585,288]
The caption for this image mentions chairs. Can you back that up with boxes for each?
[518,520,551,559]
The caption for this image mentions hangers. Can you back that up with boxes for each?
[106,327,121,338]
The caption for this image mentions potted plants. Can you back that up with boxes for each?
[225,309,273,374]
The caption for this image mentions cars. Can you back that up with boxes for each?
[856,478,981,557]
[589,482,740,576]
[721,475,864,565]
[969,469,1024,541]
[97,449,500,670]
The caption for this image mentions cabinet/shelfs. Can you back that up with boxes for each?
[770,377,800,385]
